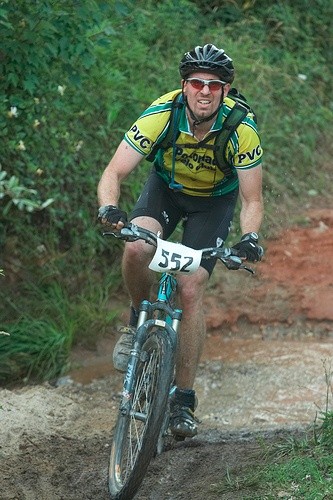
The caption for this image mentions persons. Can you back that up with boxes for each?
[96,43,263,436]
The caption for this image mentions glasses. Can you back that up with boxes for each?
[186,78,226,91]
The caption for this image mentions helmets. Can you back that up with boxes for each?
[179,43,234,83]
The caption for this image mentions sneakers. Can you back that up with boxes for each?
[170,388,198,440]
[110,328,138,371]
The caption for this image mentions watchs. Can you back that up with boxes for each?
[242,232,259,243]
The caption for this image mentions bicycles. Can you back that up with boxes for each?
[96,211,255,499]
